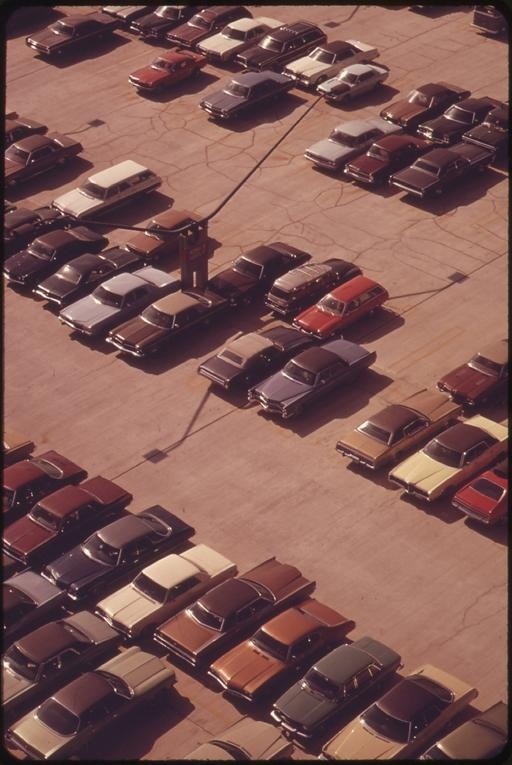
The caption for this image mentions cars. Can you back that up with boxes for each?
[0,131,84,195]
[2,205,72,262]
[19,9,121,62]
[126,210,209,265]
[165,4,254,49]
[343,131,435,190]
[317,663,479,760]
[303,114,405,176]
[1,644,177,763]
[267,635,404,752]
[0,570,72,652]
[130,5,212,43]
[280,38,381,90]
[58,264,183,340]
[337,339,507,534]
[48,158,163,224]
[153,555,317,675]
[93,543,241,644]
[128,48,209,94]
[1,438,37,467]
[1,608,121,723]
[207,597,356,713]
[315,61,389,106]
[247,339,378,421]
[40,503,196,606]
[379,78,472,129]
[460,102,512,162]
[385,141,496,204]
[31,246,142,309]
[101,4,160,26]
[415,699,510,760]
[2,449,89,530]
[196,324,321,393]
[1,112,49,151]
[197,69,294,125]
[206,240,313,310]
[2,225,110,289]
[1,475,133,568]
[292,274,389,342]
[179,714,296,762]
[416,93,503,147]
[265,257,363,322]
[103,286,230,361]
[470,4,507,34]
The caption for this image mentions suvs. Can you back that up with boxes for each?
[233,20,328,75]
